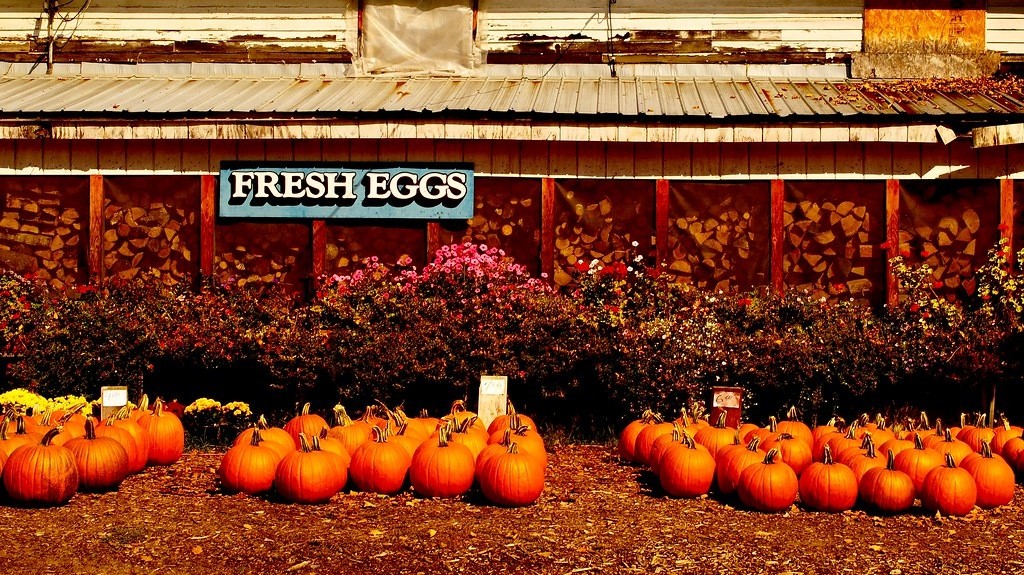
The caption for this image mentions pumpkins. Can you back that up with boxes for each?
[1,393,184,506]
[619,402,1024,514]
[220,398,548,505]
